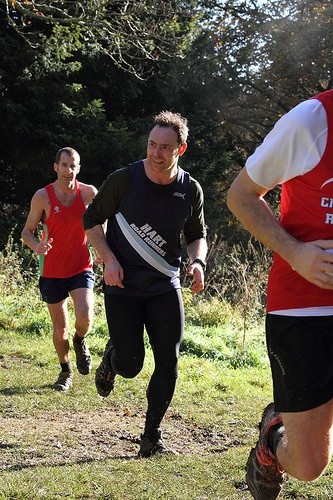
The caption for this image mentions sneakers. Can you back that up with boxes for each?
[52,370,74,391]
[139,431,180,459]
[73,339,92,374]
[244,401,286,500]
[95,338,118,396]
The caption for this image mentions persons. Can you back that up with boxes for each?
[226,88,333,500]
[22,147,109,391]
[82,111,208,461]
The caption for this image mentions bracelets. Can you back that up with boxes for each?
[191,259,207,273]
